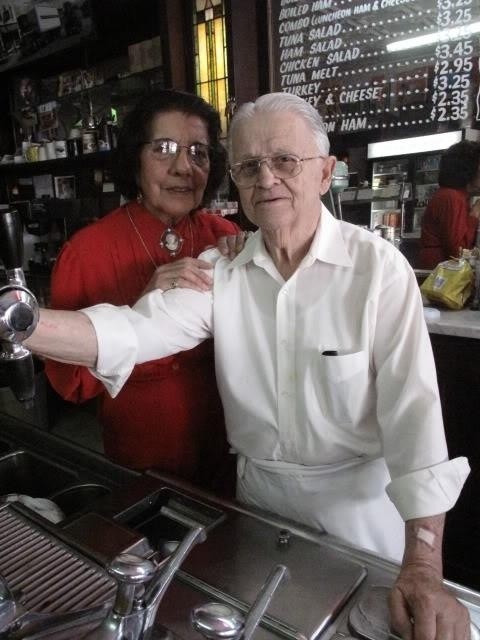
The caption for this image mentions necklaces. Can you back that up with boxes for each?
[126,199,197,276]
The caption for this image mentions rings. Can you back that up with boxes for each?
[170,279,178,290]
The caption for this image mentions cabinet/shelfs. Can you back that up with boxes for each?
[0,19,167,288]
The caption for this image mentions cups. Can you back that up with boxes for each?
[1,101,98,164]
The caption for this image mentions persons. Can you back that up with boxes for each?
[40,84,246,499]
[164,232,180,250]
[10,87,472,639]
[421,136,479,268]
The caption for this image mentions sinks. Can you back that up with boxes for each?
[0,429,15,457]
[0,448,79,504]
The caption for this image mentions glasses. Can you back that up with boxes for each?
[141,137,212,159]
[230,154,326,188]
